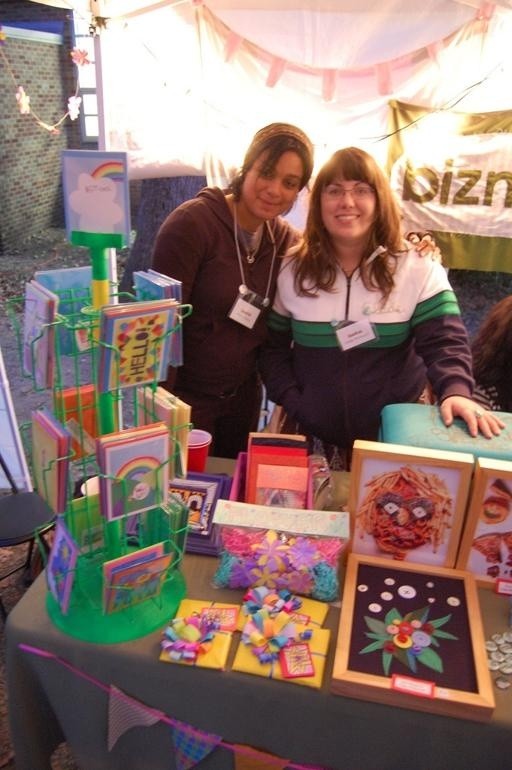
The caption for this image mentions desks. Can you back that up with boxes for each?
[9,456,510,770]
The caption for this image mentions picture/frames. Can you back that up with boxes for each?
[331,553,496,723]
[351,440,474,570]
[124,470,233,556]
[455,457,511,592]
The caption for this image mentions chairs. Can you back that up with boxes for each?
[1,453,58,621]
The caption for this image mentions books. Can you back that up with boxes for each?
[246,431,310,514]
[62,149,130,245]
[22,266,192,617]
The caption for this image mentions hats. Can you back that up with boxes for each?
[248,120,314,176]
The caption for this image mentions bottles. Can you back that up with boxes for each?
[307,454,334,507]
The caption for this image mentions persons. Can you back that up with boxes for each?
[154,122,314,470]
[255,147,507,470]
[420,297,512,416]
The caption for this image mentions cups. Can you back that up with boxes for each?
[186,430,212,474]
